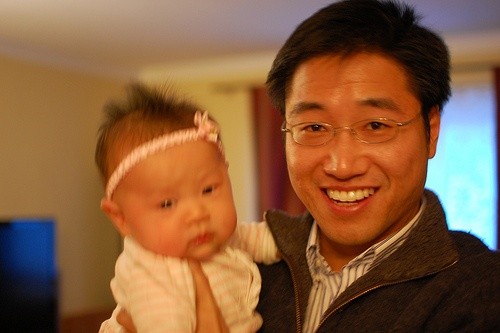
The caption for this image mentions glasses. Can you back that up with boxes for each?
[280,107,427,146]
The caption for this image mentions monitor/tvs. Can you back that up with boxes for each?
[0,219,58,332]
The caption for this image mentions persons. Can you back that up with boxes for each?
[94,79,283,333]
[116,0,500,333]
